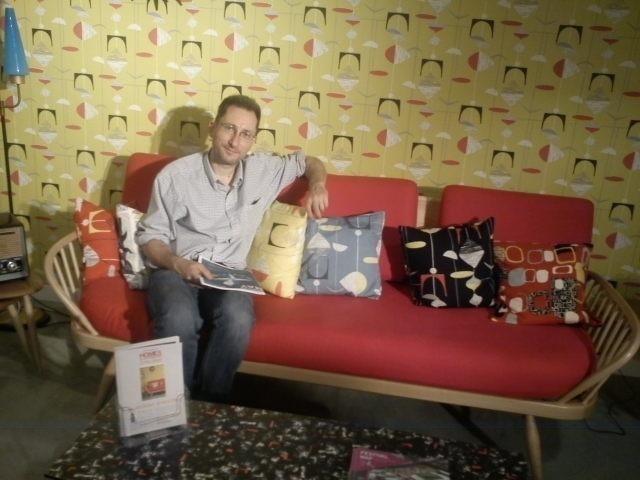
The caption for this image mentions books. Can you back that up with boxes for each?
[349,447,451,479]
[114,335,187,437]
[197,253,267,296]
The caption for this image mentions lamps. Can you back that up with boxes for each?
[1,8,49,331]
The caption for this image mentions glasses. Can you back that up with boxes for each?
[220,121,254,140]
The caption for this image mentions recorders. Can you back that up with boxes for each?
[0,212,30,282]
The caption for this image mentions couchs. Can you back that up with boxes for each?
[44,153,639,478]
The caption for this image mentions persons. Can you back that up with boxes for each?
[222,269,254,289]
[137,95,328,405]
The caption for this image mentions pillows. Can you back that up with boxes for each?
[489,242,601,327]
[296,210,385,299]
[398,216,495,308]
[115,203,150,290]
[246,200,308,300]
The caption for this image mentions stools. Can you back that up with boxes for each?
[1,272,46,373]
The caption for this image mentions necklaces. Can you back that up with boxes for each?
[215,164,234,179]
